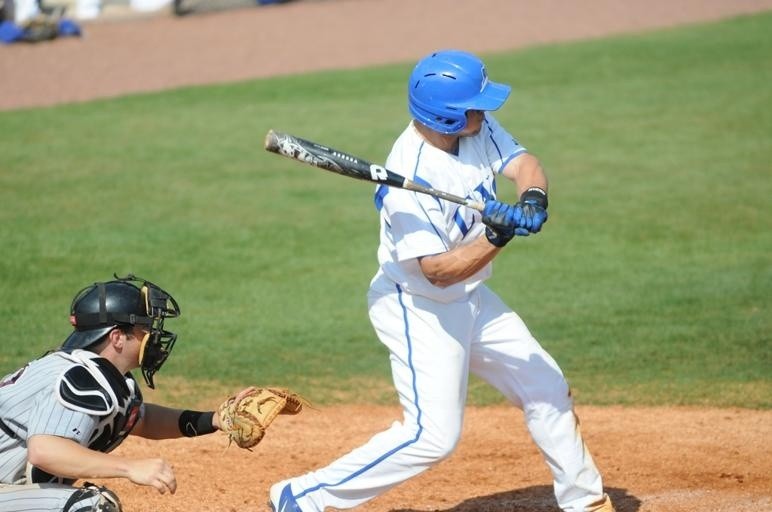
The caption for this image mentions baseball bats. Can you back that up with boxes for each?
[264,129,534,228]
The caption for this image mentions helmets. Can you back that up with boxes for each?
[58,269,181,390]
[406,47,514,136]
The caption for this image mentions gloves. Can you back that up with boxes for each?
[480,185,550,248]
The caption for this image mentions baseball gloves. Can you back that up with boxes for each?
[218,387,317,457]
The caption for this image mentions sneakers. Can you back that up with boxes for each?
[266,477,304,512]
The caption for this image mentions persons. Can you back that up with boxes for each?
[269,48,617,512]
[0,273,302,512]
